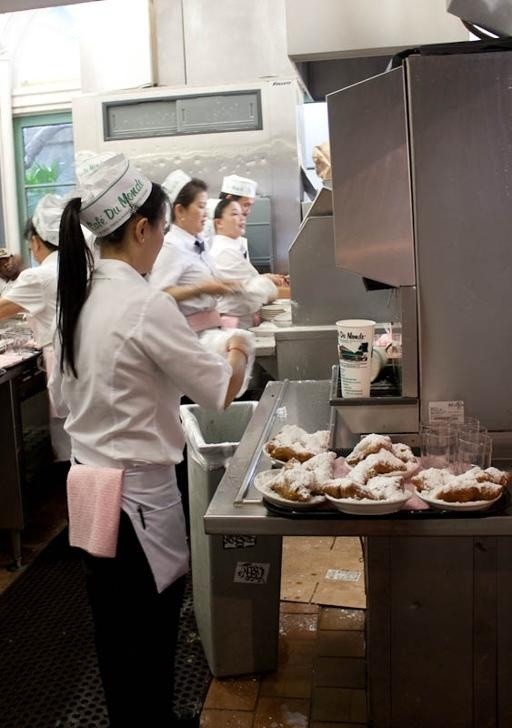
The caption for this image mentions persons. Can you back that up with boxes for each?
[147,169,244,337]
[198,199,279,328]
[1,194,82,467]
[0,248,25,281]
[43,156,250,728]
[204,174,286,288]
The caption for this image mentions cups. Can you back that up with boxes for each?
[370,346,388,382]
[419,414,493,474]
[335,318,378,398]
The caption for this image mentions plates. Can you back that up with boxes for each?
[272,314,293,328]
[411,487,503,512]
[345,458,419,480]
[262,442,286,467]
[259,305,284,321]
[252,468,326,512]
[272,298,290,304]
[324,490,413,517]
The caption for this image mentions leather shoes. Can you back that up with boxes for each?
[119,706,200,725]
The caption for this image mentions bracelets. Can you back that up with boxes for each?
[227,343,250,364]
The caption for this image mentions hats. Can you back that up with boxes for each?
[202,197,223,234]
[221,174,260,199]
[33,192,67,245]
[73,149,123,187]
[61,155,153,237]
[161,168,191,210]
[0,247,16,261]
